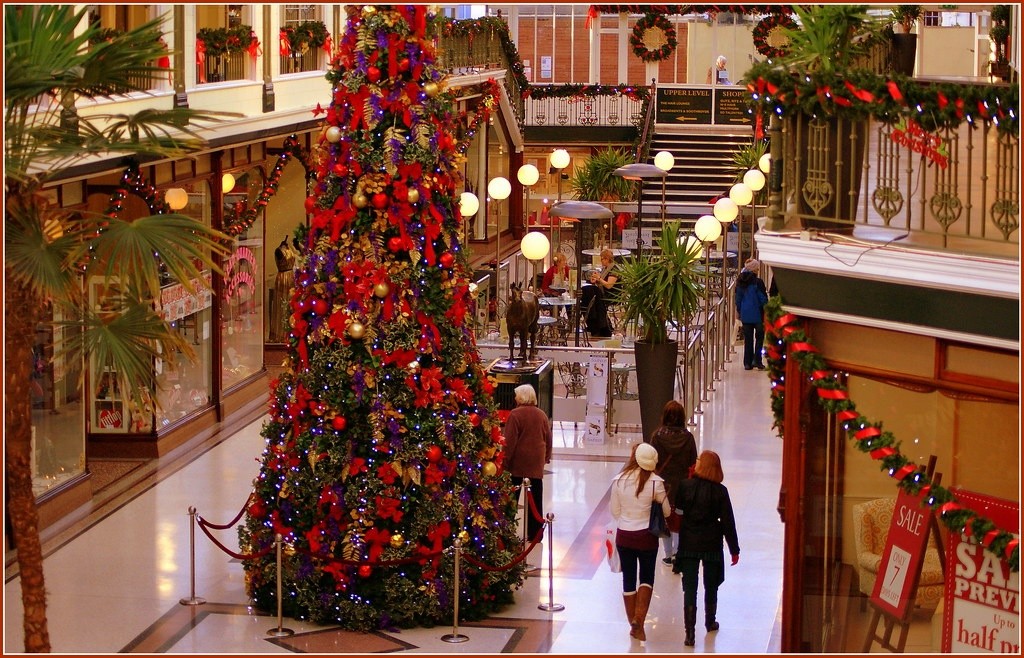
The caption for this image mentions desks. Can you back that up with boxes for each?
[702,248,738,265]
[537,316,559,345]
[586,364,637,395]
[538,295,577,318]
[581,247,632,258]
[691,265,718,275]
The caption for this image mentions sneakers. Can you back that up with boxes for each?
[661,557,671,565]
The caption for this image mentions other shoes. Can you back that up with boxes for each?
[745,365,753,370]
[752,359,766,369]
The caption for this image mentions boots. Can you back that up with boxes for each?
[630,583,653,641]
[684,605,697,646]
[705,602,719,632]
[622,591,636,638]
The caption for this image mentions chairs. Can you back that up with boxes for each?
[540,295,716,434]
[852,496,945,625]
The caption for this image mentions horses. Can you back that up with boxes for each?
[507,281,540,366]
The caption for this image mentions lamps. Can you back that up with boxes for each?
[165,188,190,212]
[223,173,236,194]
[40,216,64,240]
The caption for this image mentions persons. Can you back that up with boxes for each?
[542,254,570,320]
[706,56,727,84]
[674,451,740,645]
[610,443,671,640]
[502,384,552,543]
[735,259,768,370]
[651,400,697,575]
[585,249,621,332]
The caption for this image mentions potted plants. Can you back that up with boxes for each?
[600,216,711,443]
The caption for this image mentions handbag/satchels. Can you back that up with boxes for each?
[730,319,745,346]
[602,519,624,573]
[648,481,672,538]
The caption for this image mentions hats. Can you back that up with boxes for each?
[745,259,760,271]
[635,443,658,470]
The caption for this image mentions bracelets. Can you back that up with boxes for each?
[599,277,601,279]
